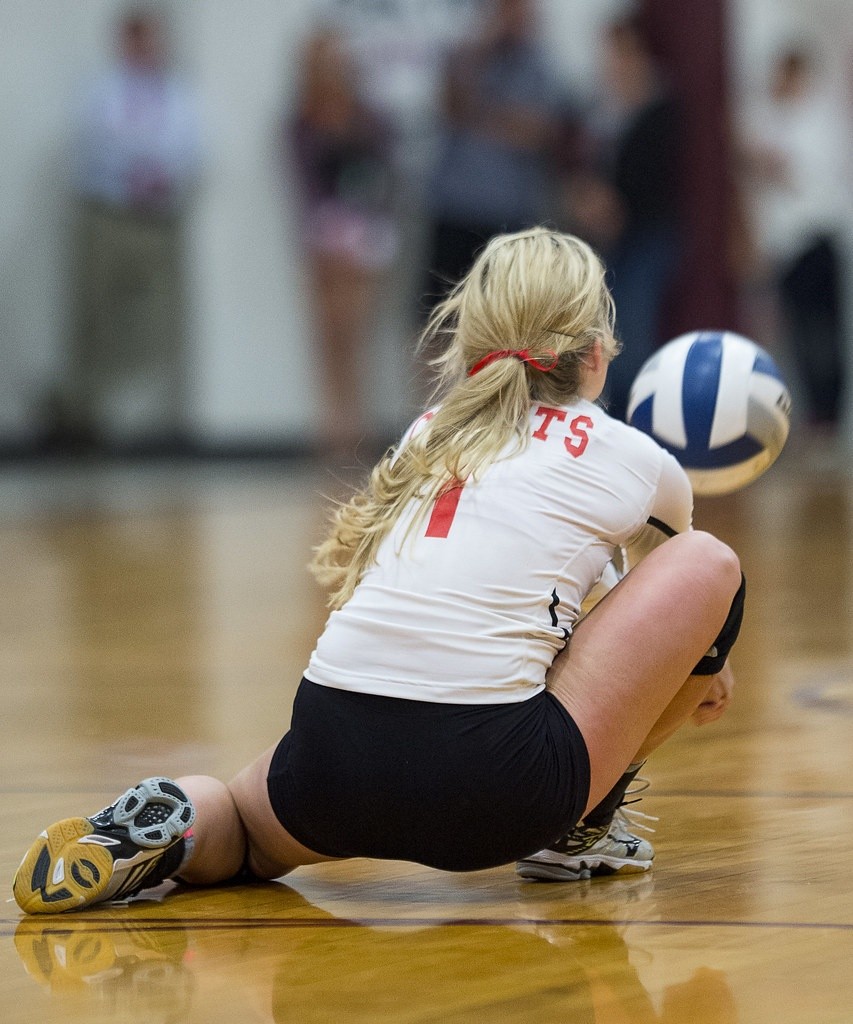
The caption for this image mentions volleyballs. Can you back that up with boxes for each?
[625,328,794,496]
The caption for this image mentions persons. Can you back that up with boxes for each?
[21,3,846,470]
[15,228,748,911]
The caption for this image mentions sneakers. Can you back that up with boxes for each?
[12,776,196,915]
[515,777,659,882]
